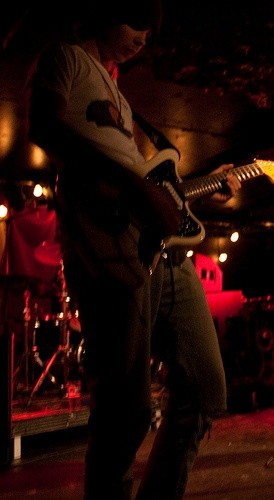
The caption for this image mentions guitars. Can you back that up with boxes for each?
[115,157,274,269]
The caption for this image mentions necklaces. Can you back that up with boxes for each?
[82,46,124,127]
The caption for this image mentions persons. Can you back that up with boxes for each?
[27,0,241,500]
